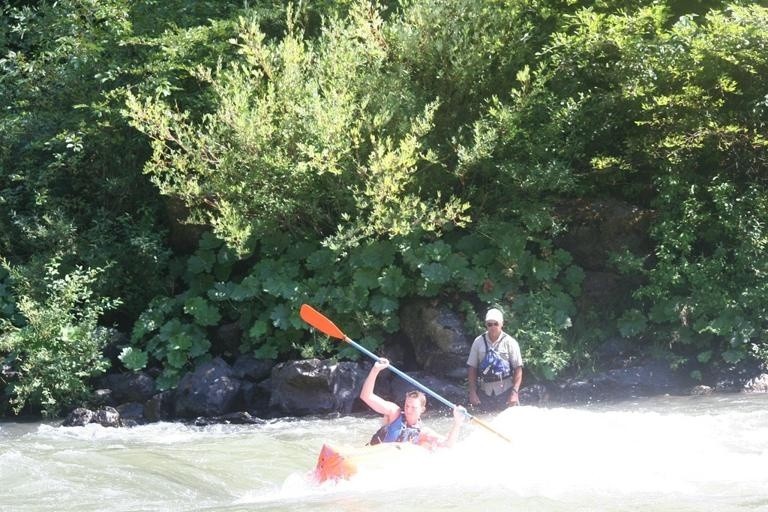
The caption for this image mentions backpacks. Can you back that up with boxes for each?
[478,333,511,380]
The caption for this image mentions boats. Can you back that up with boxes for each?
[311,438,429,488]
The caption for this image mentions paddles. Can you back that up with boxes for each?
[299,304,510,441]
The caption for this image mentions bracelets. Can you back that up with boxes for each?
[513,387,518,393]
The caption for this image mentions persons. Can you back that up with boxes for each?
[360,358,466,449]
[466,308,523,413]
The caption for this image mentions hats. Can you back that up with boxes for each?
[485,306,506,325]
